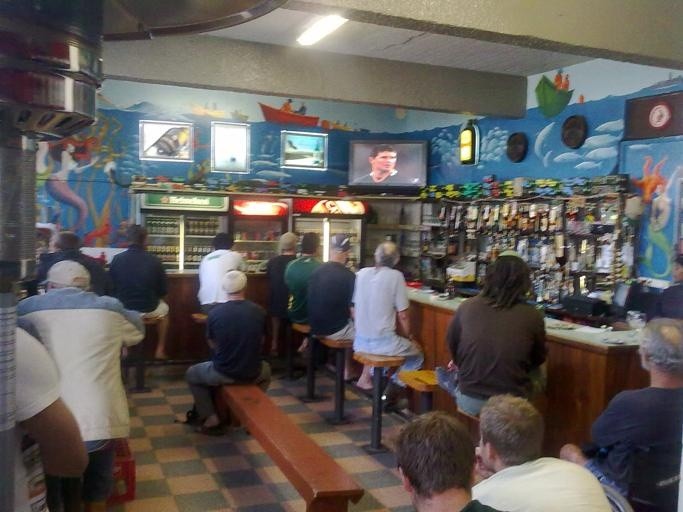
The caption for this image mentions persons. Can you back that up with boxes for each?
[391,410,497,512]
[198,232,248,315]
[468,393,612,512]
[347,241,425,414]
[16,260,145,512]
[560,319,683,500]
[19,232,108,296]
[16,325,90,512]
[661,254,683,318]
[351,144,419,185]
[267,231,320,356]
[185,270,272,437]
[109,225,172,360]
[437,256,549,416]
[308,233,375,390]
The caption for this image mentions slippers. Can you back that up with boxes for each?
[194,420,226,437]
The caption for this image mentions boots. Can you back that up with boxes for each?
[381,382,409,412]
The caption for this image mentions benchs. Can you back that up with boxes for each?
[212,382,364,511]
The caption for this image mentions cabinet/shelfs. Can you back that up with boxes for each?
[136,195,229,268]
[290,199,367,271]
[230,197,290,259]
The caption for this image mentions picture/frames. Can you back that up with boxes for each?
[209,121,250,175]
[280,130,329,170]
[138,120,194,162]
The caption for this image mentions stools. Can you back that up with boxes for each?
[351,353,405,454]
[322,338,354,426]
[129,319,156,393]
[399,371,443,416]
[293,323,323,402]
[191,313,207,324]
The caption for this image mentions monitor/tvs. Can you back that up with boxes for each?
[348,140,428,194]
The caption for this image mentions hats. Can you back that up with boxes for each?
[330,235,351,251]
[222,271,248,293]
[279,232,297,250]
[37,259,91,289]
